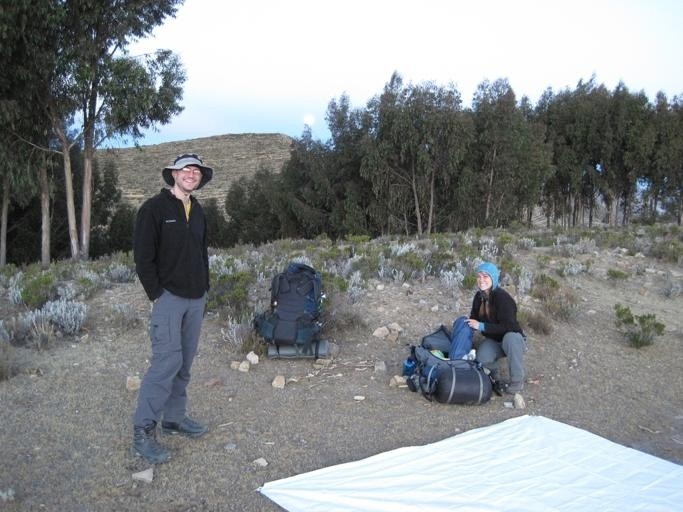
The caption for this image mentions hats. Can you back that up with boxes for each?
[476,263,499,290]
[162,153,213,190]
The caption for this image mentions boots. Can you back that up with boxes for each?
[161,419,210,438]
[134,424,173,464]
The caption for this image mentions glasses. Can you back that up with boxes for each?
[183,167,201,174]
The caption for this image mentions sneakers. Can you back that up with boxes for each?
[507,381,524,395]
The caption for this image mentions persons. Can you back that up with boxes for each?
[131,152,213,464]
[463,262,528,396]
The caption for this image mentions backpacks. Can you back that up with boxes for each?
[403,322,493,405]
[254,262,325,359]
